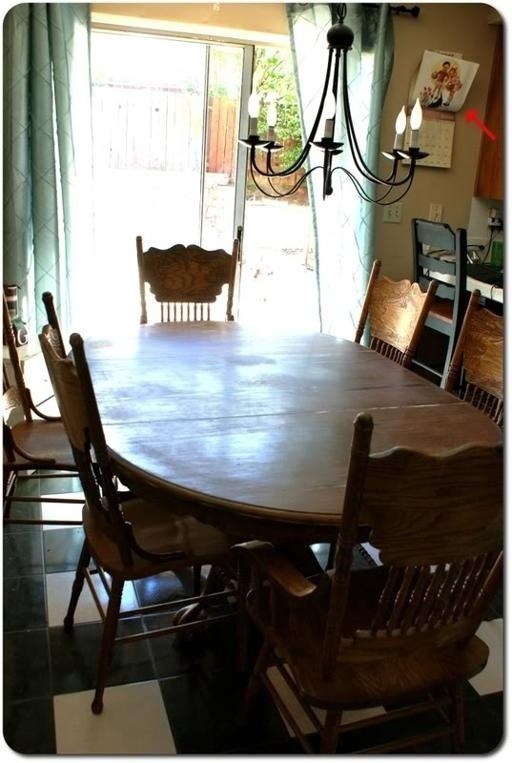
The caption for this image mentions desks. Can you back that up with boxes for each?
[63,317,503,674]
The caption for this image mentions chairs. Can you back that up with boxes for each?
[2,283,254,715]
[353,215,505,432]
[175,410,503,756]
[135,232,241,325]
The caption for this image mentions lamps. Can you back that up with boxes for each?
[232,3,437,206]
